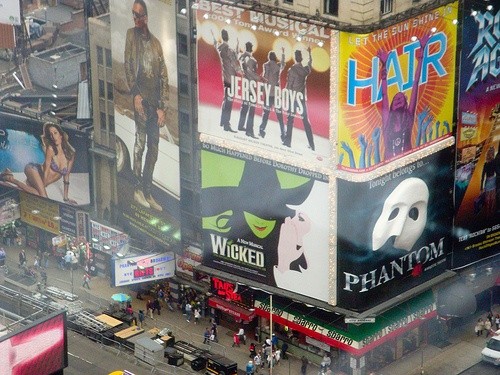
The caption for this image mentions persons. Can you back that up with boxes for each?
[477,312,500,337]
[0,220,93,288]
[376,32,432,159]
[318,351,335,375]
[211,28,315,151]
[246,333,289,375]
[126,279,208,328]
[202,320,219,345]
[300,356,308,375]
[124,0,169,211]
[0,123,77,206]
[231,326,246,348]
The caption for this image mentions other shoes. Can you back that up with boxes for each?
[282,142,291,147]
[258,134,264,138]
[225,127,237,133]
[134,189,150,209]
[238,127,246,131]
[146,194,162,212]
[246,132,259,139]
[308,147,315,151]
[220,123,224,126]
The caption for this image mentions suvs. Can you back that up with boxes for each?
[480,328,500,367]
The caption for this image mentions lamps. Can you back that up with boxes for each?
[50,81,77,121]
[90,233,181,271]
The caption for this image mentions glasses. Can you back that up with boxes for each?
[132,9,145,18]
